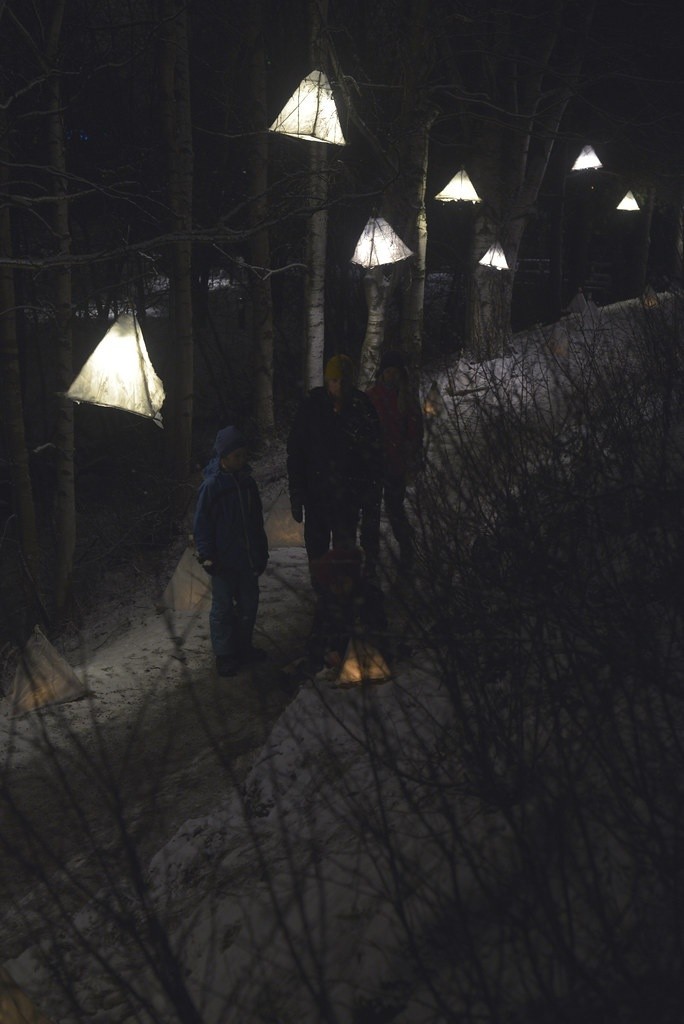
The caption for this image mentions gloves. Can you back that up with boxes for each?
[288,470,309,523]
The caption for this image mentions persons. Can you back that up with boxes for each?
[288,351,424,614]
[195,426,269,676]
[282,549,387,680]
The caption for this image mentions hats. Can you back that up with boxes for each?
[372,351,398,378]
[216,426,248,459]
[321,562,355,578]
[325,354,355,380]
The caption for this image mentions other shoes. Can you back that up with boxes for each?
[234,657,253,671]
[214,653,239,678]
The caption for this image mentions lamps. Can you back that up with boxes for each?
[56,66,661,412]
[0,622,86,723]
[155,483,314,620]
[420,378,445,420]
[333,617,393,687]
